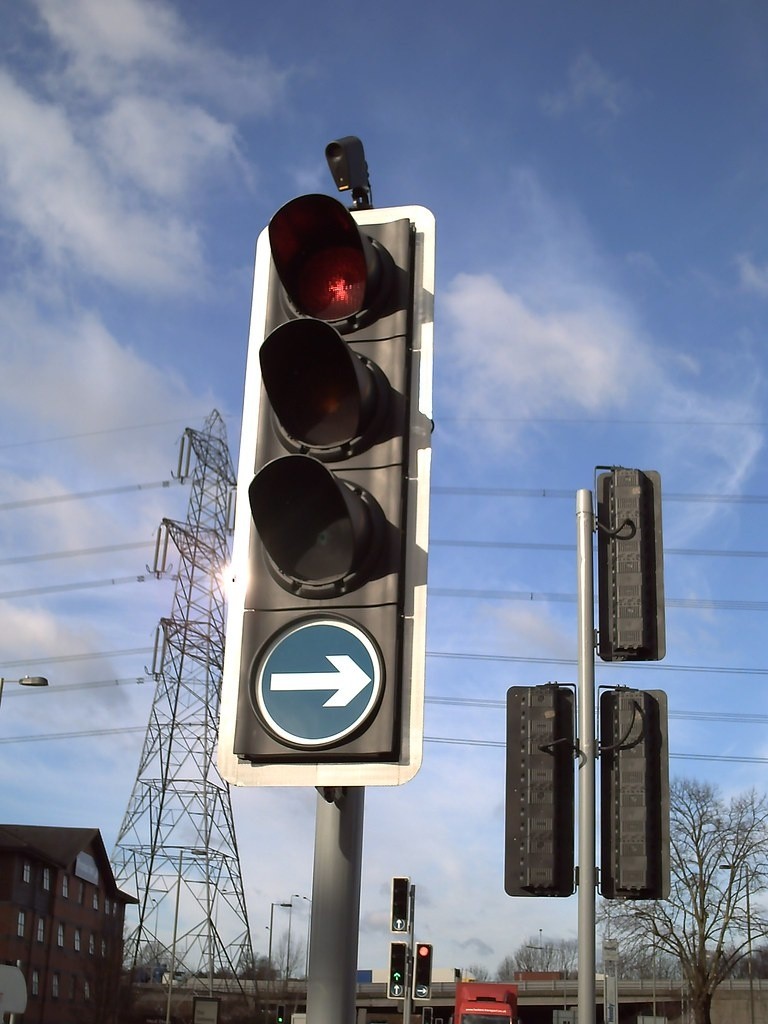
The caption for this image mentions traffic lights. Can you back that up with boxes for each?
[217,193,434,784]
[276,1005,285,1024]
[391,876,411,933]
[412,942,433,1000]
[387,941,409,999]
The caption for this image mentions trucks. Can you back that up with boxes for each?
[455,981,519,1024]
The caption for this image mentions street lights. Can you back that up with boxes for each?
[265,903,292,1024]
[303,896,311,981]
[0,676,49,707]
[719,865,755,1024]
[152,899,158,964]
[165,850,208,1024]
[526,945,567,1012]
[539,929,543,946]
[285,895,299,991]
